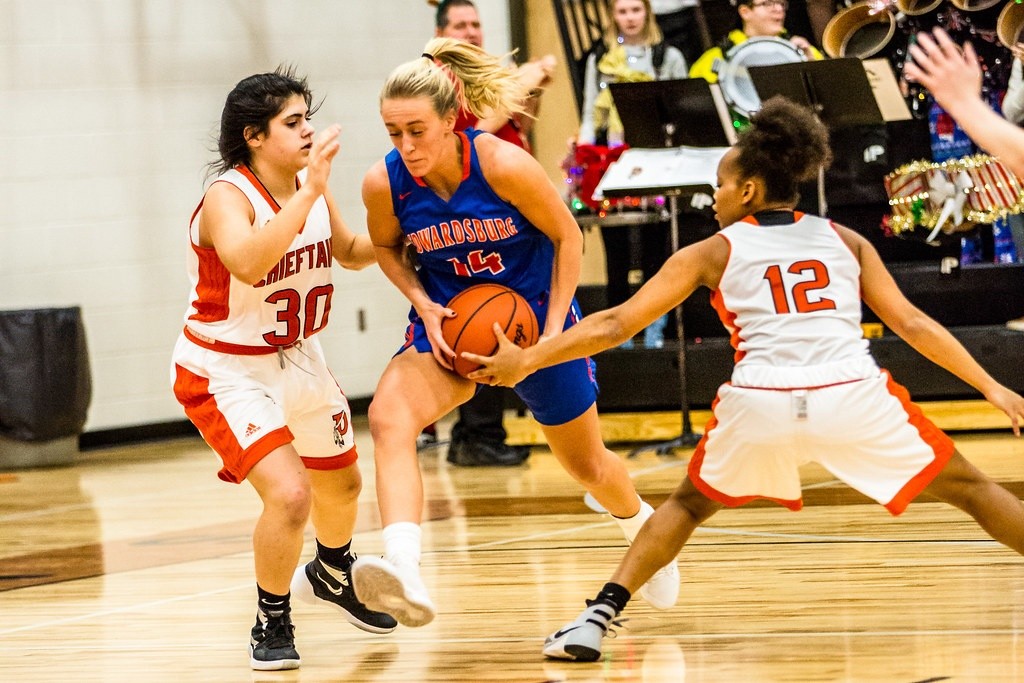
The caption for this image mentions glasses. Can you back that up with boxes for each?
[751,0,789,8]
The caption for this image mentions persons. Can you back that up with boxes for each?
[351,37,681,627]
[434,0,556,468]
[460,94,1024,662]
[904,27,1024,188]
[576,0,686,146]
[686,0,825,81]
[170,60,398,671]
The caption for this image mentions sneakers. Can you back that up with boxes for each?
[541,592,620,662]
[247,605,301,671]
[351,555,435,629]
[624,501,680,611]
[297,555,398,635]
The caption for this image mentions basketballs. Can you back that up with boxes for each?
[439,282,539,384]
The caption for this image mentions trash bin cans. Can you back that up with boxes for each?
[0,305,93,468]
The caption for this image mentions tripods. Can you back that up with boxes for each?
[597,195,704,460]
